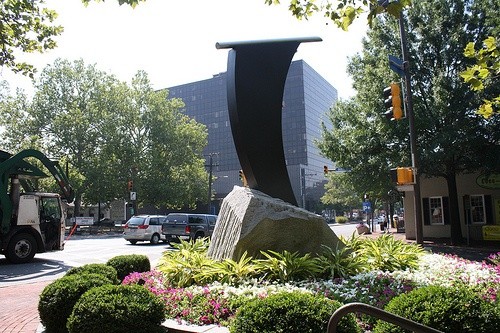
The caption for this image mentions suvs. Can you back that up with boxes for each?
[161,213,218,246]
[122,214,167,245]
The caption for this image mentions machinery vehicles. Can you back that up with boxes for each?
[0,147,76,263]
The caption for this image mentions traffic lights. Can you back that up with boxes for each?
[390,167,413,185]
[128,181,133,190]
[363,193,369,199]
[382,82,402,120]
[324,166,328,177]
[238,170,245,181]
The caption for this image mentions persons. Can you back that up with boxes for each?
[397,211,404,217]
[380,214,388,231]
